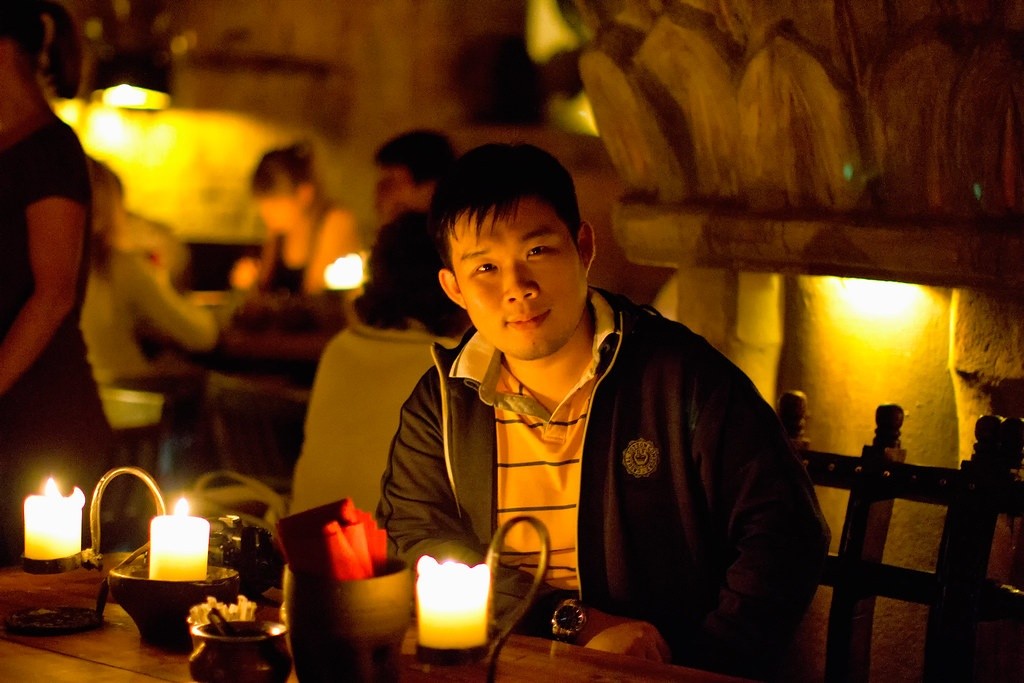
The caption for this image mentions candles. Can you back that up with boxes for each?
[149,497,210,581]
[23,478,85,560]
[413,554,490,649]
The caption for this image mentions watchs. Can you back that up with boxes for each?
[551,598,586,644]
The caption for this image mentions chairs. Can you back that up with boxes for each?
[775,389,1024,683]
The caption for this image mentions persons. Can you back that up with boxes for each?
[375,143,831,683]
[80,128,459,517]
[0,0,123,573]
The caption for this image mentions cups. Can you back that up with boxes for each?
[283,557,412,683]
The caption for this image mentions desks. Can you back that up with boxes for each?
[0,551,741,683]
